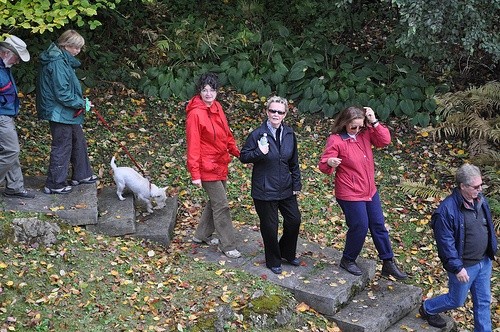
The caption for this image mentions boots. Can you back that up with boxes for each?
[381,259,408,280]
[339,257,362,276]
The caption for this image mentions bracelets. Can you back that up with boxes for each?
[371,119,379,125]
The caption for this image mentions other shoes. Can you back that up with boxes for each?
[72,174,97,186]
[271,267,282,274]
[217,246,241,258]
[281,256,301,266]
[192,236,219,246]
[44,185,72,194]
[419,303,446,328]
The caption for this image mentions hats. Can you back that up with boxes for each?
[0,35,31,62]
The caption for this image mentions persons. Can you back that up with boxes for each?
[36,30,100,194]
[0,36,34,199]
[319,106,408,280]
[238,96,301,274]
[419,162,498,332]
[185,72,242,259]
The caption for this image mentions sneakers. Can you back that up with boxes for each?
[4,188,35,199]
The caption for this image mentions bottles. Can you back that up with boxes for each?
[260,133,268,147]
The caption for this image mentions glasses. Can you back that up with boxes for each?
[347,123,366,130]
[268,108,286,115]
[468,182,485,190]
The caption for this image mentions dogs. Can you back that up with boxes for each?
[108,155,170,213]
